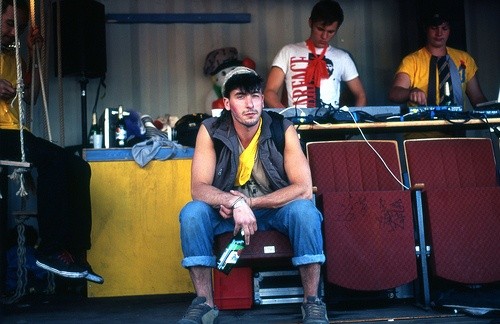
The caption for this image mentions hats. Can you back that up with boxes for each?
[222,66,258,97]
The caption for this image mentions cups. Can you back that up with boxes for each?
[93,131,103,149]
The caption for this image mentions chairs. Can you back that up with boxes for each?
[216,138,500,324]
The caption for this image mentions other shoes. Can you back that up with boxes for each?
[36,249,104,285]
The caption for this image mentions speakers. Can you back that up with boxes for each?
[52,0,107,79]
[399,0,467,59]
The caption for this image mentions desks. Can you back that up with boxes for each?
[82,146,196,298]
[294,118,500,173]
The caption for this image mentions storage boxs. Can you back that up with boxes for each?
[212,267,253,310]
[253,269,325,305]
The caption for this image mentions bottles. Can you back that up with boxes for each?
[114,106,128,147]
[215,227,250,277]
[88,110,101,146]
[440,81,456,107]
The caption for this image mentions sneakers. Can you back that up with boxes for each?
[177,296,218,324]
[301,295,329,324]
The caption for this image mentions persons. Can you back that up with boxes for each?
[0,0,104,282]
[177,65,327,323]
[388,19,489,106]
[264,0,365,107]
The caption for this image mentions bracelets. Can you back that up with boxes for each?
[230,195,243,209]
[250,197,251,208]
